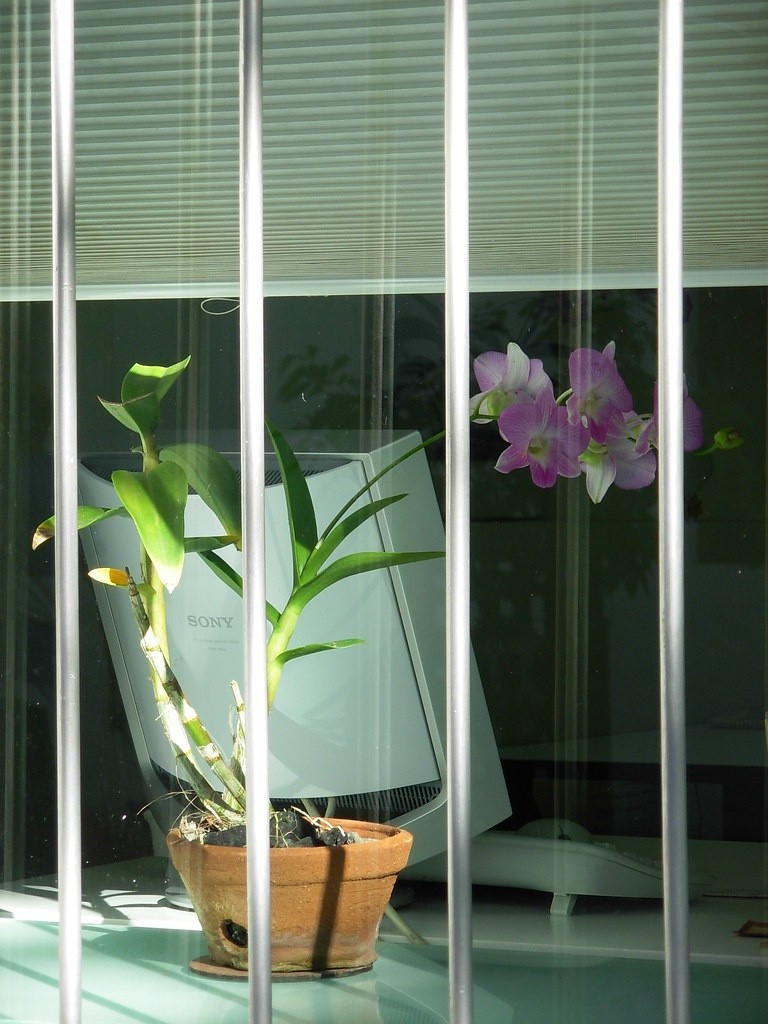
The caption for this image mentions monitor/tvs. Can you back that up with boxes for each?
[77,427,514,910]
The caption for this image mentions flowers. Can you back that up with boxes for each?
[33,340,742,846]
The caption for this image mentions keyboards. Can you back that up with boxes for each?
[396,834,705,918]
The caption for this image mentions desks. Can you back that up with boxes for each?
[497,719,768,842]
[0,834,768,1024]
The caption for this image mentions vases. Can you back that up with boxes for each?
[165,817,412,977]
